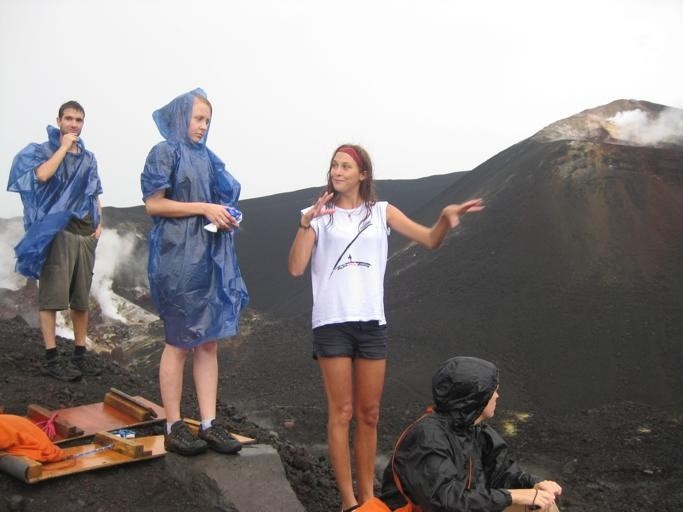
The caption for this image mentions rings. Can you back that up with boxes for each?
[218,220,223,225]
[222,216,226,220]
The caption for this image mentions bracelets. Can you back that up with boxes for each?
[298,220,310,229]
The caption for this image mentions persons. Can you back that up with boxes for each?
[140,88,248,456]
[377,356,562,512]
[6,99,103,382]
[287,143,486,511]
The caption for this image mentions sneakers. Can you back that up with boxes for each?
[42,356,82,381]
[163,420,210,457]
[70,357,102,376]
[198,419,242,454]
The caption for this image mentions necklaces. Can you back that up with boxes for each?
[336,203,361,217]
